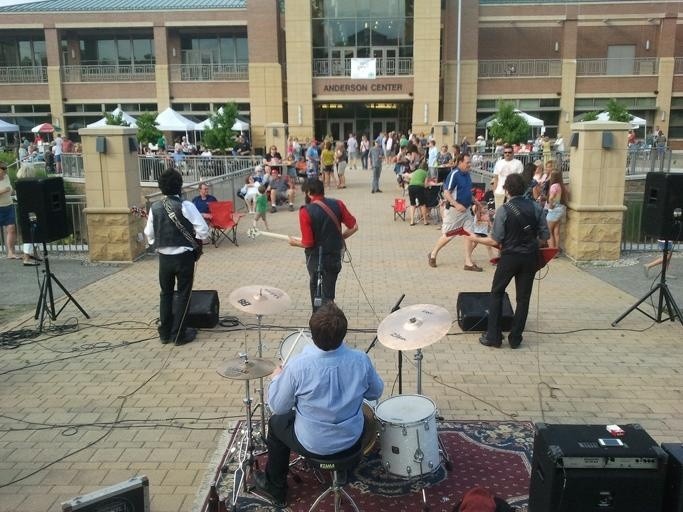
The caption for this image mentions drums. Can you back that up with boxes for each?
[375,394,440,480]
[280,330,313,368]
[362,397,377,456]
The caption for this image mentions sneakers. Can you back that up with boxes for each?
[159,333,171,344]
[173,334,193,346]
[428,253,437,268]
[288,205,294,211]
[270,206,277,213]
[463,263,483,273]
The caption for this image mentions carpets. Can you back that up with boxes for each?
[189,419,535,512]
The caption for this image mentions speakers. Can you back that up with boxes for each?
[639,172,683,242]
[14,174,73,244]
[457,291,516,330]
[161,289,219,327]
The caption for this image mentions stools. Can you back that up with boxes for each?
[307,450,363,512]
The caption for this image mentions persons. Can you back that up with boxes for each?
[14,164,43,266]
[16,134,81,175]
[239,129,359,230]
[142,168,210,344]
[643,241,679,281]
[138,133,251,177]
[289,178,358,314]
[407,133,567,260]
[0,162,20,260]
[464,174,550,349]
[625,124,667,167]
[422,154,482,272]
[360,129,427,193]
[188,182,217,221]
[249,303,383,501]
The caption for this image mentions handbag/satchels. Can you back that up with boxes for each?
[366,150,369,152]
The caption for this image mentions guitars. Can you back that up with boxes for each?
[249,227,346,261]
[445,228,559,272]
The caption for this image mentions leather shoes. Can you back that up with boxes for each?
[479,336,502,348]
[508,333,523,349]
[29,252,43,261]
[372,189,382,193]
[252,469,288,501]
[23,258,40,266]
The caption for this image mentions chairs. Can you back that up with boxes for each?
[206,201,245,248]
[392,198,409,221]
[415,188,441,225]
[276,175,290,206]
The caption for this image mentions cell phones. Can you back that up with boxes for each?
[598,438,625,448]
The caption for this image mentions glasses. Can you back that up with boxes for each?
[504,152,512,154]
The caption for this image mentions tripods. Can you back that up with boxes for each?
[26,243,90,332]
[219,315,274,478]
[610,241,683,327]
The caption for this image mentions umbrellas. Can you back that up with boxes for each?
[30,122,62,141]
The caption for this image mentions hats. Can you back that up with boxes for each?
[271,169,279,174]
[477,135,484,140]
[311,140,318,144]
[535,160,542,166]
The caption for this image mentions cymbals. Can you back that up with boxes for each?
[216,356,276,380]
[228,284,291,315]
[377,304,452,350]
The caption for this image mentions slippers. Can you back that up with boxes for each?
[410,222,417,226]
[6,254,21,259]
[424,222,430,226]
